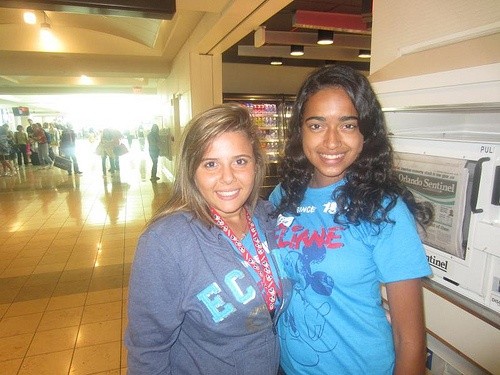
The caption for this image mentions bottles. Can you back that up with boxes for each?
[243,103,292,162]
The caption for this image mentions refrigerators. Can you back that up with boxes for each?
[223,93,297,201]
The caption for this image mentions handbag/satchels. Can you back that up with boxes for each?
[26,143,30,152]
[54,155,71,170]
[96,143,107,156]
[119,143,129,157]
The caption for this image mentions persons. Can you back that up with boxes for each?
[97,128,123,173]
[125,106,281,375]
[0,119,75,176]
[60,123,83,176]
[265,67,433,375]
[147,124,161,182]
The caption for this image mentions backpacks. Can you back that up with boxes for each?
[43,130,51,144]
[31,151,41,165]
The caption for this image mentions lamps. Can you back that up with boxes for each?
[271,57,283,64]
[317,30,333,45]
[358,50,371,58]
[290,46,305,56]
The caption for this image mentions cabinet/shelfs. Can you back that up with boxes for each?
[224,94,297,162]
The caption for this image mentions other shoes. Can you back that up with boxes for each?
[108,168,112,171]
[38,166,46,170]
[150,176,160,182]
[49,162,53,169]
[74,171,83,175]
[110,174,115,177]
[102,173,108,177]
[67,170,73,176]
[1,166,19,176]
[115,168,120,170]
